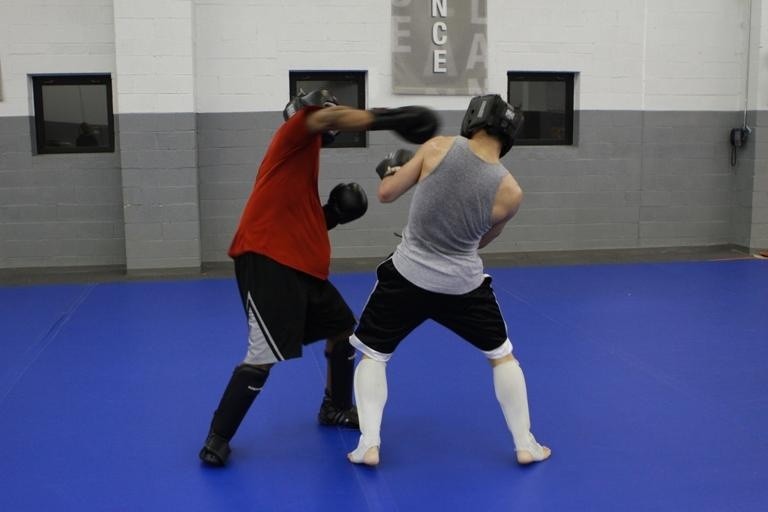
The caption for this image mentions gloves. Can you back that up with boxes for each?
[375,149,415,180]
[322,182,368,231]
[365,106,438,144]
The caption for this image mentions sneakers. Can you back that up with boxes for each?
[202,431,231,465]
[317,401,361,429]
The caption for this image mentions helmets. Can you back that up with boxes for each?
[460,94,522,158]
[283,88,343,138]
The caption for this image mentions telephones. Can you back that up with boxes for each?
[730,127,744,147]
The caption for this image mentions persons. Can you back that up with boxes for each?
[74,122,98,146]
[347,93,553,467]
[200,90,438,469]
